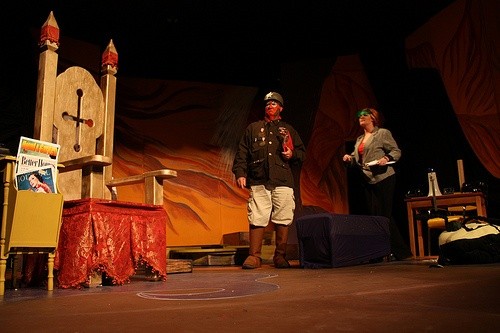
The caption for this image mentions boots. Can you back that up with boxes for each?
[242,224,264,269]
[273,223,290,268]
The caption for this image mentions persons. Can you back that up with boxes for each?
[28,172,52,194]
[231,91,306,269]
[343,107,414,262]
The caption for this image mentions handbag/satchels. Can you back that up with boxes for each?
[438,216,500,264]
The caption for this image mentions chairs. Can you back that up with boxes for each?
[34,10,177,286]
[427,191,477,257]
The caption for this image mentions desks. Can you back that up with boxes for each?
[0,155,66,295]
[405,191,488,262]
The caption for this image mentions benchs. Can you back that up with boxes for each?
[296,214,391,267]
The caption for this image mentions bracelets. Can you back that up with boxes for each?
[384,156,389,161]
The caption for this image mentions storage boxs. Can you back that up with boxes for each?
[223,231,274,246]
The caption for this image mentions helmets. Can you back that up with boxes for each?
[264,92,284,106]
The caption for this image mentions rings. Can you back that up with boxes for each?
[287,155,289,157]
